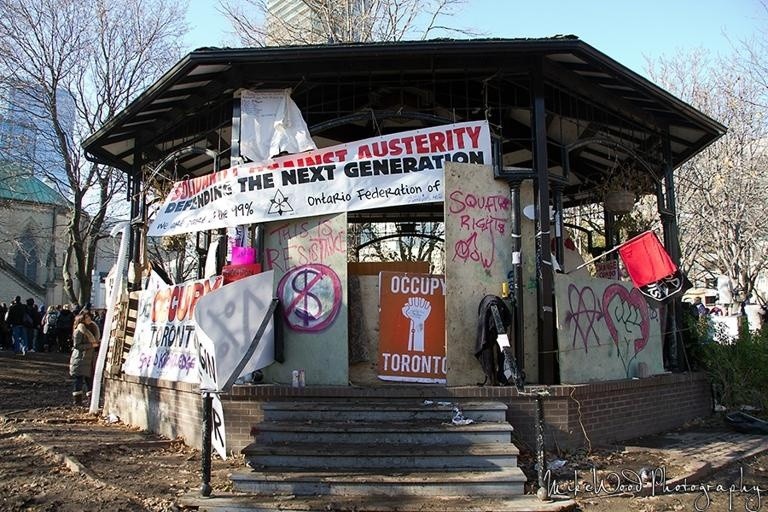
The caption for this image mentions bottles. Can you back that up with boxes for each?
[292,368,305,388]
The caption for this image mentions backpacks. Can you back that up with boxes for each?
[43,311,58,335]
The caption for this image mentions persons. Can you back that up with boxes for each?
[0,296,107,408]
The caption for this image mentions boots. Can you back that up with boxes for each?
[86,391,92,403]
[72,391,84,406]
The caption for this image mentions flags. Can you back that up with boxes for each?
[618,231,677,290]
